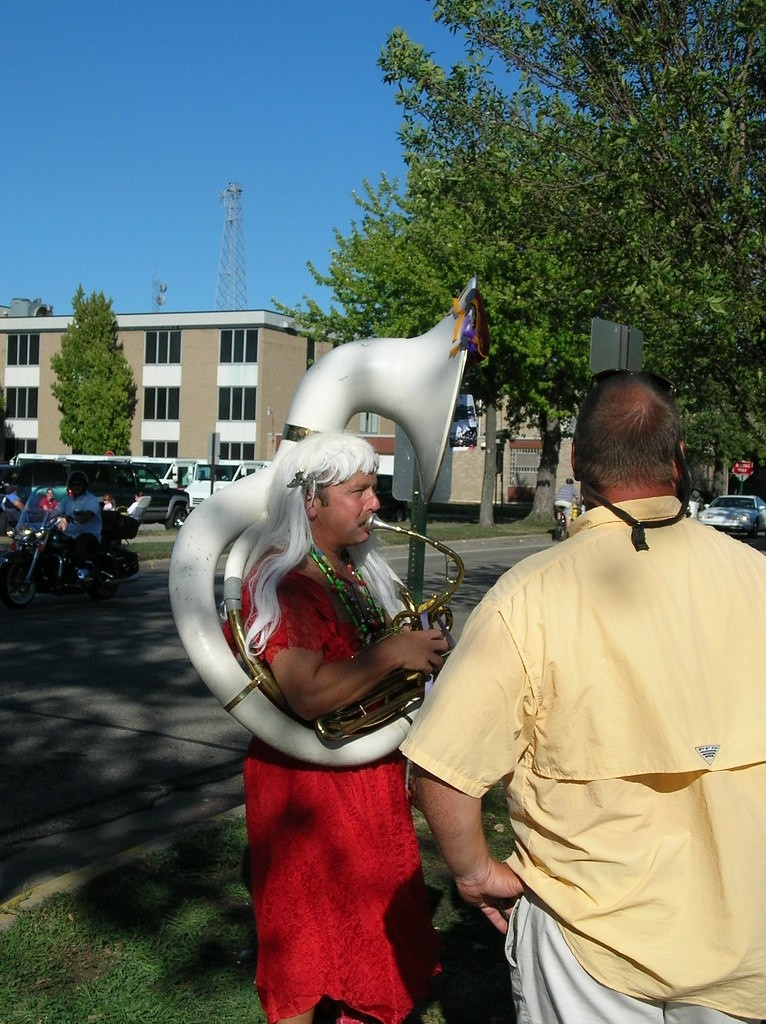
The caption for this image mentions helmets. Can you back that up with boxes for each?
[566,478,575,484]
[67,470,89,487]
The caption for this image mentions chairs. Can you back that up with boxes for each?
[126,495,151,534]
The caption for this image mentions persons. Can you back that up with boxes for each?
[1,484,60,516]
[101,491,152,515]
[551,474,577,537]
[221,432,449,1024]
[48,470,101,581]
[399,367,766,1023]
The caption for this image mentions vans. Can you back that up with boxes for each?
[4,459,190,530]
[10,452,273,514]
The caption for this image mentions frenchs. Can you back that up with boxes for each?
[167,271,474,769]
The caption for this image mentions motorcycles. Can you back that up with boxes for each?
[1,508,140,607]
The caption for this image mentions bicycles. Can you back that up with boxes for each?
[555,506,569,542]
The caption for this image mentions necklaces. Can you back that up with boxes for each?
[308,546,384,651]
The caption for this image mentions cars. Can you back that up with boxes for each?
[696,495,766,539]
